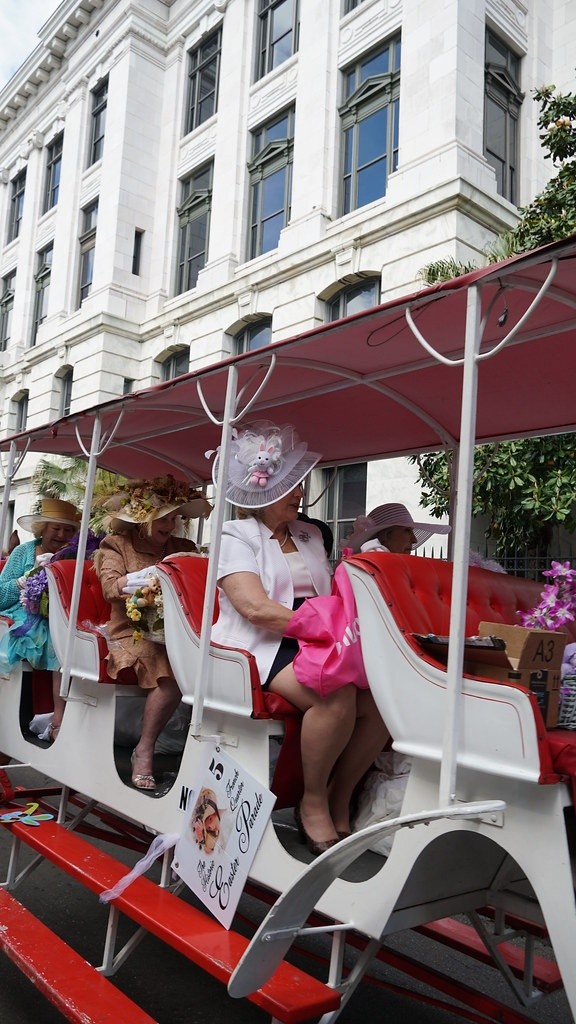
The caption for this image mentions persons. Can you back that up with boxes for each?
[336,502,452,555]
[93,475,213,789]
[200,416,390,856]
[0,497,81,742]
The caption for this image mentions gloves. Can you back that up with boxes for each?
[127,565,157,585]
[33,553,54,572]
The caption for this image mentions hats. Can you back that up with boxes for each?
[17,498,82,532]
[204,419,324,508]
[340,503,451,554]
[88,474,216,537]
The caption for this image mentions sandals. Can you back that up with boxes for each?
[49,722,60,740]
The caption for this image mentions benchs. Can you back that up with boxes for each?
[45,559,138,685]
[0,560,32,671]
[342,552,576,785]
[155,556,303,719]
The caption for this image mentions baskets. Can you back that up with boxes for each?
[557,676,576,729]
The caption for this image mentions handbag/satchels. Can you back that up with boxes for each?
[281,548,370,698]
[351,752,414,858]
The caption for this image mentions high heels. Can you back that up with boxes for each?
[293,799,350,855]
[130,749,156,789]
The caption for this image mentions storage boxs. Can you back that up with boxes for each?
[409,621,567,729]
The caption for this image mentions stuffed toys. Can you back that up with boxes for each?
[247,441,276,486]
[135,579,162,608]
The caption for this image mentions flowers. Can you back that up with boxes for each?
[20,566,49,614]
[515,561,576,632]
[126,582,164,645]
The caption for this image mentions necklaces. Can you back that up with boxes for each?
[41,544,47,553]
[159,547,166,559]
[279,528,288,548]
